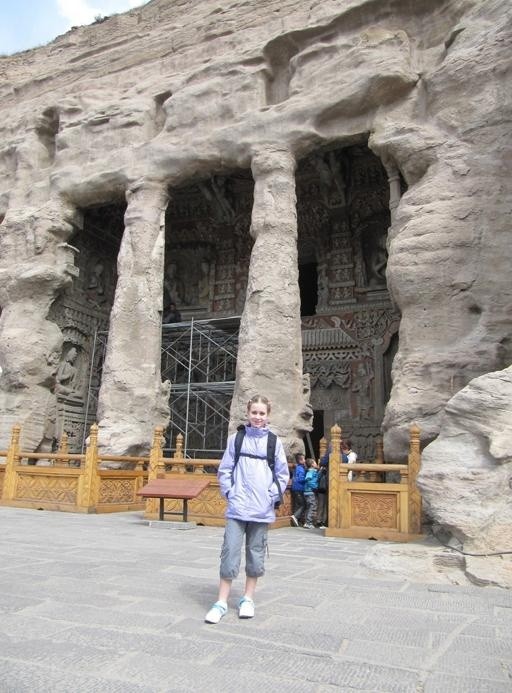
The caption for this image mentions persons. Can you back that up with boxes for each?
[55,346,83,398]
[163,302,183,324]
[340,437,358,481]
[304,457,325,529]
[291,451,307,526]
[200,392,291,624]
[86,263,108,307]
[196,259,213,305]
[318,440,348,528]
[162,261,185,306]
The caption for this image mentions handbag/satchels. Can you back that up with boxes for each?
[317,466,327,494]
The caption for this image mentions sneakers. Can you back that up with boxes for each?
[238,596,255,618]
[203,600,228,623]
[290,513,327,530]
